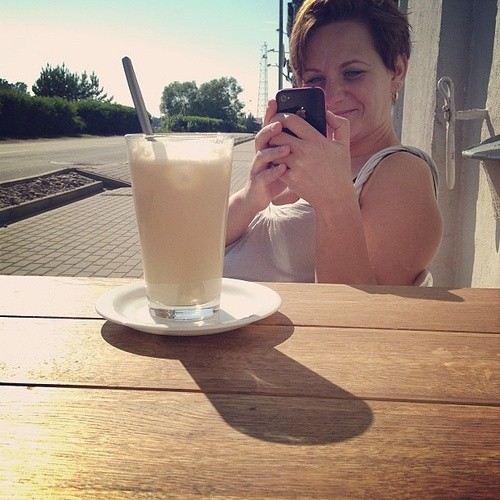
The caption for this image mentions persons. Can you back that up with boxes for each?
[222,0,444,286]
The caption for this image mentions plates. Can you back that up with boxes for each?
[95,277,281,336]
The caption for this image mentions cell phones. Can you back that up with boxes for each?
[276,87,327,139]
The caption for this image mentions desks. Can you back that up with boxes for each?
[0,276,500,500]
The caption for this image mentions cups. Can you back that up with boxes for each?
[126,132,236,321]
